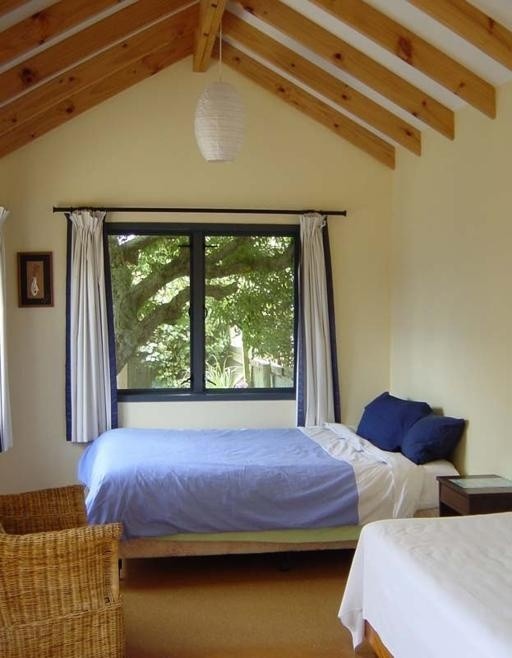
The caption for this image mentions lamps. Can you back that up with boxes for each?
[195,15,244,163]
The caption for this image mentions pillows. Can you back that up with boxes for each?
[401,413,466,465]
[356,392,433,454]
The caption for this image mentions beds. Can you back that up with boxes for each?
[336,510,512,657]
[75,422,461,579]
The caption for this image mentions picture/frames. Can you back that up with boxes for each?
[15,248,54,309]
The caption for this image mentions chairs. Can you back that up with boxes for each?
[0,482,128,658]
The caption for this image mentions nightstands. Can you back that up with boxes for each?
[436,473,512,517]
